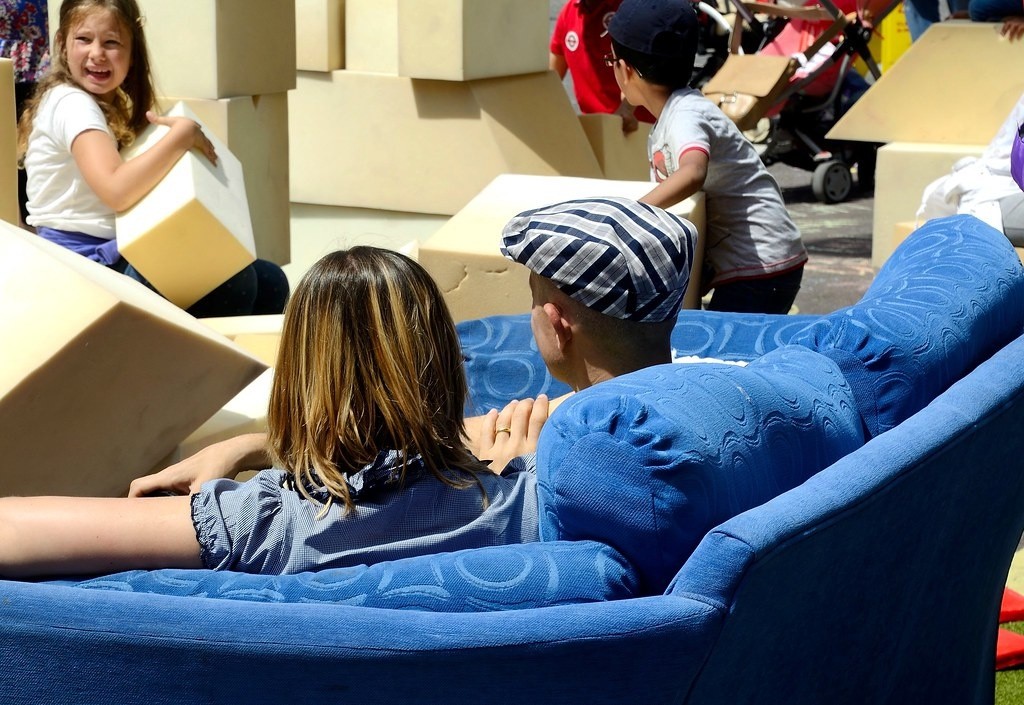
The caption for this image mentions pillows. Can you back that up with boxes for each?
[39,539,640,613]
[535,343,864,595]
[788,214,1024,439]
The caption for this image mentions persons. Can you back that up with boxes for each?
[0,195,750,581]
[550,0,808,315]
[17,0,290,319]
[903,0,1024,44]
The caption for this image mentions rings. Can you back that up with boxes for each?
[495,427,511,435]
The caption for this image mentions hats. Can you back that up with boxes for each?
[609,0,700,53]
[498,195,697,325]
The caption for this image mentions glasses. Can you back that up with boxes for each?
[603,52,643,78]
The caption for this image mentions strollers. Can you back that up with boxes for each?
[722,0,889,203]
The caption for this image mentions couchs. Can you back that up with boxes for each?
[0,309,1024,705]
